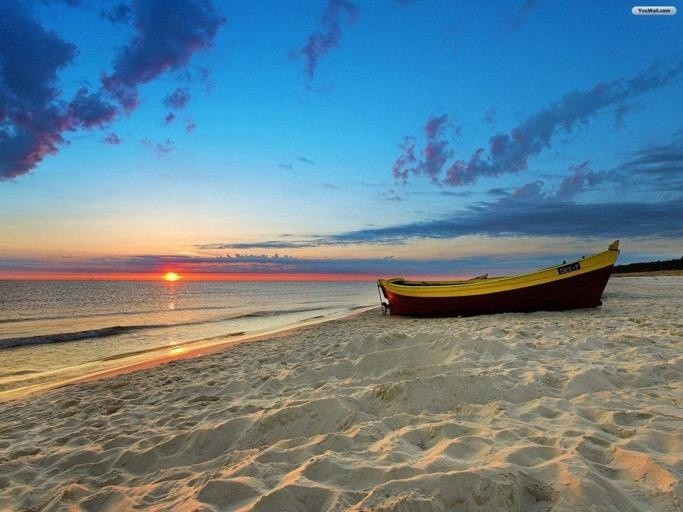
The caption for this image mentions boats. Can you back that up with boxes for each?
[375,240,620,315]
